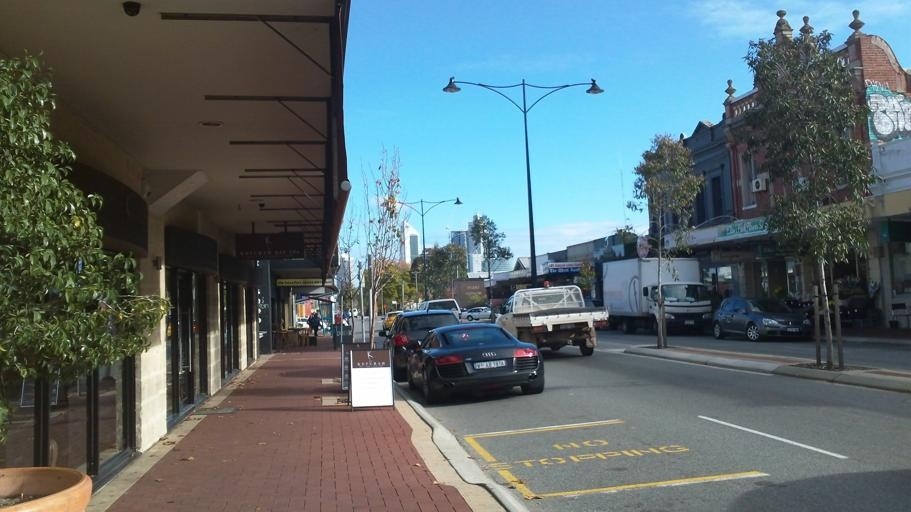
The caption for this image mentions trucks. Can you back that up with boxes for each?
[602,258,713,334]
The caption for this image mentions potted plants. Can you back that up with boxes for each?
[0,44,177,512]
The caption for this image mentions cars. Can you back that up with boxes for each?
[167,360,188,395]
[324,308,359,326]
[461,307,502,320]
[381,310,405,330]
[408,321,545,403]
[713,296,812,341]
[297,317,327,331]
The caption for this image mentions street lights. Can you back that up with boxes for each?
[443,77,604,284]
[404,270,427,295]
[382,196,463,300]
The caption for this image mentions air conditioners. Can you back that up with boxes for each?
[751,176,766,193]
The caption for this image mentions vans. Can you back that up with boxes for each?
[418,298,463,322]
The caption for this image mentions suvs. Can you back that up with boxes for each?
[379,309,460,383]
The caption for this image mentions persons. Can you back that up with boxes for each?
[306,313,322,337]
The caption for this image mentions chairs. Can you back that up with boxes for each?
[273,327,311,350]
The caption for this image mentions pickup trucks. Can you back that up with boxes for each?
[495,281,609,357]
